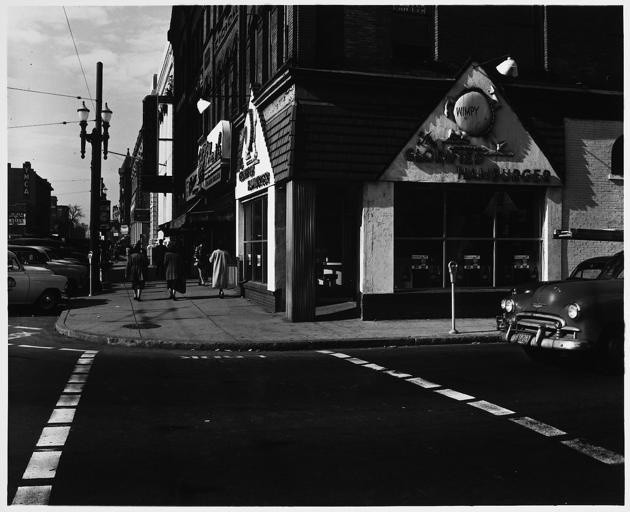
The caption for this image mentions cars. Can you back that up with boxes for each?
[497,251,622,374]
[7,236,88,310]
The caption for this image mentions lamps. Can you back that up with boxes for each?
[474,52,518,78]
[196,94,251,114]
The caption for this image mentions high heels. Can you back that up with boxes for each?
[170,295,176,300]
[219,292,224,299]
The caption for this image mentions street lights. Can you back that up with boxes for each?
[79,100,111,296]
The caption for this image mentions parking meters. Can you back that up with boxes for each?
[449,261,458,333]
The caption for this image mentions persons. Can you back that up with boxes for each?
[155,240,167,265]
[192,241,209,285]
[125,247,148,302]
[208,241,231,300]
[162,242,185,301]
